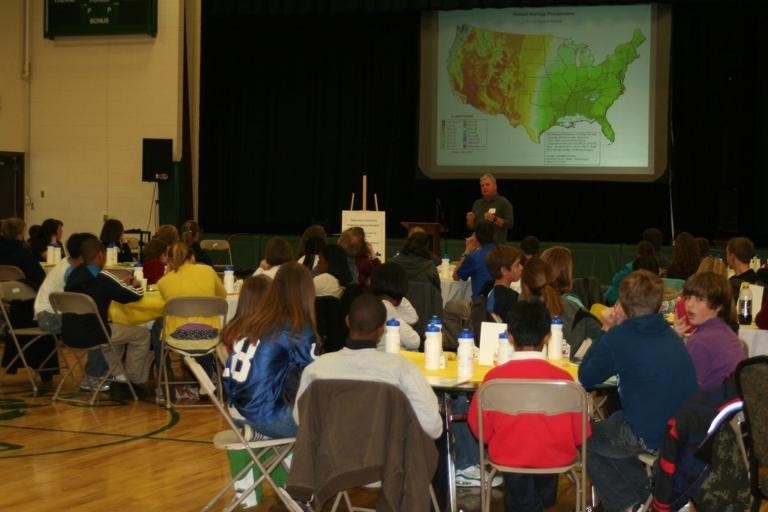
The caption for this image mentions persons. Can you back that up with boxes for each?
[466,173,514,246]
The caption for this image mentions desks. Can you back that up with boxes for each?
[402,348,581,390]
[436,264,524,306]
[739,325,767,359]
[227,285,240,321]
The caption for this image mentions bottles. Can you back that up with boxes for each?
[375,251,381,261]
[132,260,144,280]
[497,330,515,365]
[45,242,62,264]
[223,266,235,293]
[103,241,119,267]
[547,316,563,362]
[457,328,475,380]
[385,317,400,351]
[441,252,450,278]
[738,282,755,325]
[423,322,442,372]
[562,339,571,362]
[429,315,442,331]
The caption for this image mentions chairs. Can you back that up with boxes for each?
[639,400,767,512]
[737,355,768,512]
[0,266,227,411]
[182,353,298,512]
[477,379,587,512]
[329,481,440,512]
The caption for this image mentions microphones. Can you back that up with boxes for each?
[435,198,439,223]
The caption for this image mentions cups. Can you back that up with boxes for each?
[661,299,675,323]
[140,278,148,294]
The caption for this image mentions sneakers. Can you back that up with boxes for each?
[79,374,109,392]
[454,461,504,490]
[240,423,266,446]
[111,380,146,399]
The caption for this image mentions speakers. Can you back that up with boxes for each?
[143,138,172,182]
